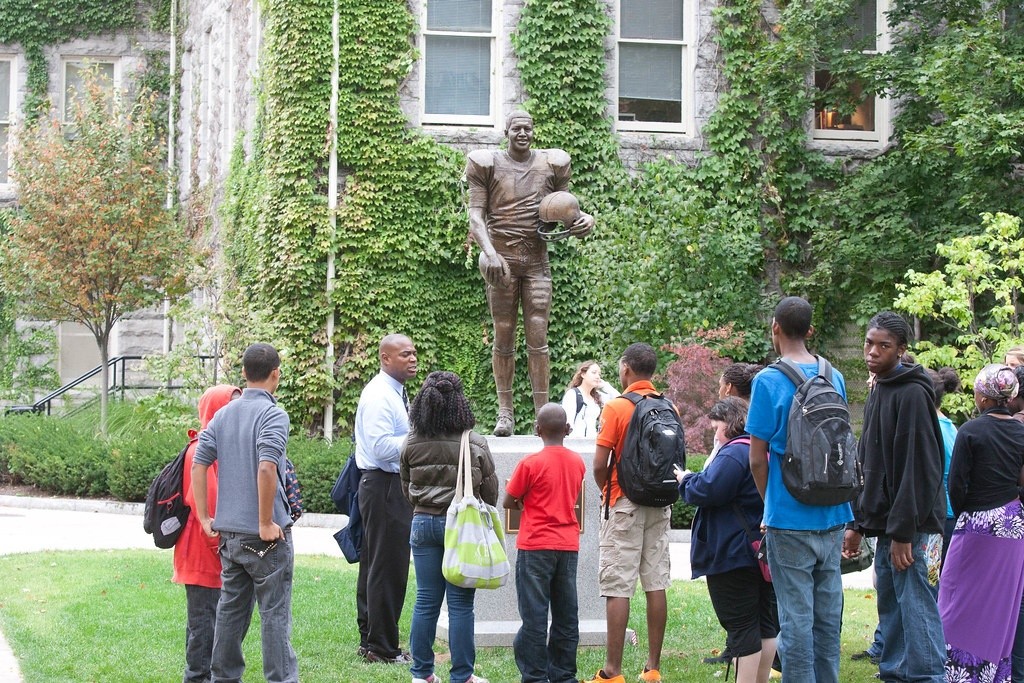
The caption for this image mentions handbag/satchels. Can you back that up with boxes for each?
[838,532,875,574]
[441,429,511,589]
[331,456,362,516]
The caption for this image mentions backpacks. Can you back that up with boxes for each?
[768,353,862,506]
[608,392,687,506]
[142,438,209,548]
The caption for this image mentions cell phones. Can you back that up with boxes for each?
[673,463,683,471]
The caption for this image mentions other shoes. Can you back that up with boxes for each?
[582,669,625,683]
[638,666,661,683]
[467,673,489,683]
[412,673,441,683]
[852,651,872,661]
[357,646,412,664]
[749,533,772,584]
[702,648,731,665]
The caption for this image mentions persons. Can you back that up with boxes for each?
[671,361,785,683]
[399,371,498,683]
[561,360,622,440]
[465,111,596,437]
[354,334,419,665]
[937,363,1024,683]
[191,343,304,683]
[503,402,585,683]
[579,342,681,683]
[745,297,851,683]
[171,384,243,683]
[1004,346,1024,683]
[842,311,962,683]
